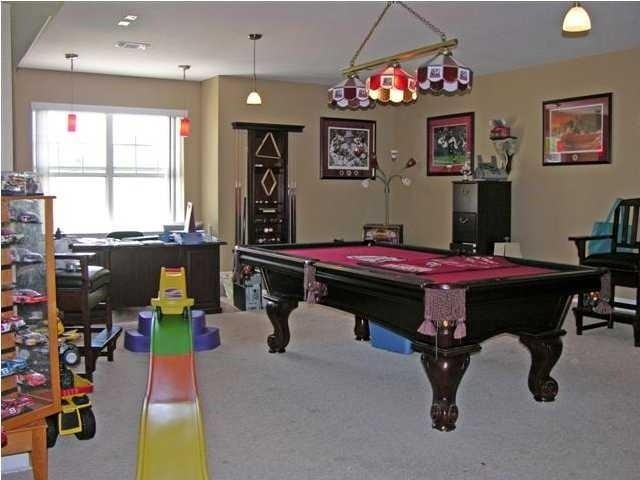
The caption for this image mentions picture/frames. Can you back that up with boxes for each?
[319,116,377,180]
[426,111,475,176]
[541,92,613,167]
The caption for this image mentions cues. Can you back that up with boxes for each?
[288,133,297,243]
[231,129,249,246]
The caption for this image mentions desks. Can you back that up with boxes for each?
[68,231,228,314]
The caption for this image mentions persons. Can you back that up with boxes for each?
[438,131,466,163]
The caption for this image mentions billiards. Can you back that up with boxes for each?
[243,265,252,273]
[587,291,600,306]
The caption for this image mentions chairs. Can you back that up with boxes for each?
[568,198,640,347]
[55,252,123,383]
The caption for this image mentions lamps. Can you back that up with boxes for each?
[65,53,78,132]
[246,34,263,105]
[560,2,592,38]
[177,64,192,137]
[115,40,152,52]
[327,1,473,111]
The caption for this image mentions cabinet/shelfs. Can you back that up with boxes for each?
[449,180,512,254]
[230,121,307,245]
[362,223,403,243]
[2,195,61,478]
